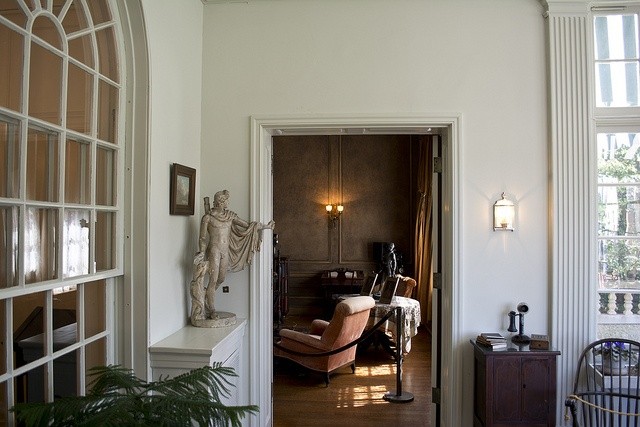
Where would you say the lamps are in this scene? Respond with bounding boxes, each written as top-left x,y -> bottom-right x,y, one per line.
326,205 -> 344,225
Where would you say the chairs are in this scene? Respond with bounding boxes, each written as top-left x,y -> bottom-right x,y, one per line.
565,338 -> 640,426
273,296 -> 375,387
395,274 -> 416,297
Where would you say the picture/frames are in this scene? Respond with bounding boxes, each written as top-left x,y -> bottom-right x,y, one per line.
360,273 -> 378,296
379,277 -> 399,304
169,163 -> 196,215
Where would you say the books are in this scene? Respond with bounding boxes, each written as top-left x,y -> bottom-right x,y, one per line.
475,332 -> 507,350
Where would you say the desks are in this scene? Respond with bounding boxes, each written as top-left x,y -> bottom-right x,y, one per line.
321,270 -> 364,319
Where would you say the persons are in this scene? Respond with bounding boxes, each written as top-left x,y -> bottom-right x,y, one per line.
195,189 -> 276,320
377,242 -> 397,296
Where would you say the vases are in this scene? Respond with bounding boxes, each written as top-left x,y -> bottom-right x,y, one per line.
603,361 -> 629,375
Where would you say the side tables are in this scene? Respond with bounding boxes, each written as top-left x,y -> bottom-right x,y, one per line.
336,294 -> 421,363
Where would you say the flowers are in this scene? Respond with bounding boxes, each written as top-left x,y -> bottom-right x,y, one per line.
593,341 -> 637,362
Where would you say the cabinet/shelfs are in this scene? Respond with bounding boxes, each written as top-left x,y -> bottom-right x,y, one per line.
470,338 -> 560,427
18,320 -> 78,407
148,318 -> 247,426
275,257 -> 289,317
